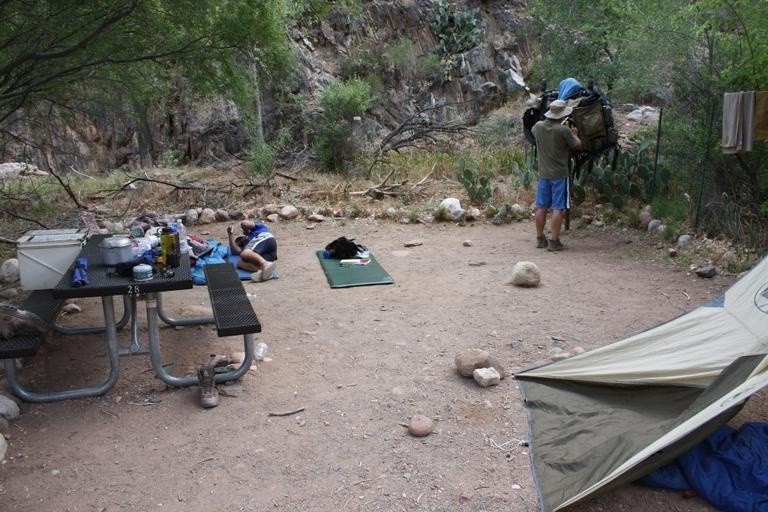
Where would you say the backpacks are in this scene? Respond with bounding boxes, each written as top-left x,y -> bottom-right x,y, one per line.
522,78 -> 623,154
325,236 -> 365,258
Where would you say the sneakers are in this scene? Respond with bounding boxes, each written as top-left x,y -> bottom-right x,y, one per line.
250,259 -> 275,283
0,303 -> 49,340
536,233 -> 569,251
196,351 -> 230,408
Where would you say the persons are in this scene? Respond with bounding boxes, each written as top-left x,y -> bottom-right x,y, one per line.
338,258 -> 373,266
531,100 -> 582,251
226,220 -> 278,281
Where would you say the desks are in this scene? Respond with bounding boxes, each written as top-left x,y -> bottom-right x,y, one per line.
3,233 -> 256,404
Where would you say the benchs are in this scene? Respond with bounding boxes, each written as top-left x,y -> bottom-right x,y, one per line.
1,262 -> 263,404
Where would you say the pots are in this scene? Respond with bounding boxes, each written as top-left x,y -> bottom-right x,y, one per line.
98,237 -> 134,266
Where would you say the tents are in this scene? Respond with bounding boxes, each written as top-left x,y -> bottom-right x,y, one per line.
514,253 -> 768,512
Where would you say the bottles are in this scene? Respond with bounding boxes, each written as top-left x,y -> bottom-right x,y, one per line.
135,235 -> 158,249
248,342 -> 269,364
176,219 -> 187,255
160,227 -> 180,268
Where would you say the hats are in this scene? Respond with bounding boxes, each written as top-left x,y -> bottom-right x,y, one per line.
543,98 -> 573,120
